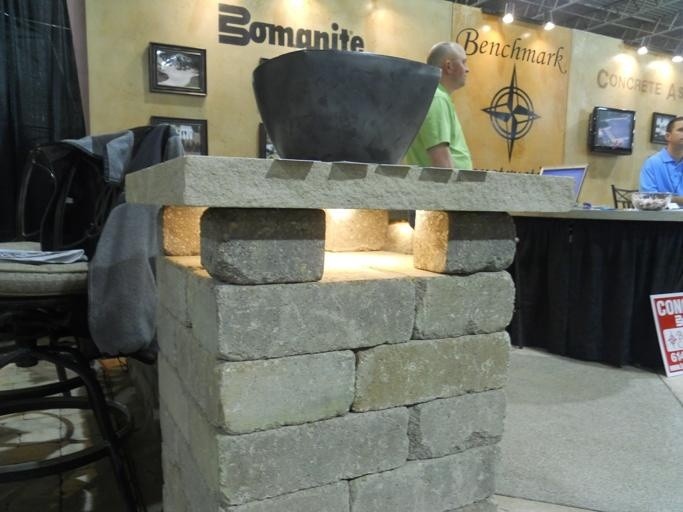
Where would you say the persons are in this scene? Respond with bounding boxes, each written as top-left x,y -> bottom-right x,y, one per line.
403,41 -> 477,171
636,116 -> 682,207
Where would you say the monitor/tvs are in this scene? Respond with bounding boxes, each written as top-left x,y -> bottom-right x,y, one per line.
589,106 -> 635,155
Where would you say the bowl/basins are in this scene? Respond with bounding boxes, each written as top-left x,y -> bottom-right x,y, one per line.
631,191 -> 673,212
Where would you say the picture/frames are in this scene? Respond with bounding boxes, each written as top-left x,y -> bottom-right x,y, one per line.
259,124 -> 277,157
151,116 -> 208,156
148,42 -> 207,95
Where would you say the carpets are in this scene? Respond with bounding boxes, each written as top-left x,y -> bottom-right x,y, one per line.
496,346 -> 682,512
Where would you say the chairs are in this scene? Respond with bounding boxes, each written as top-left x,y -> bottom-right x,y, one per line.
0,125 -> 185,512
611,185 -> 639,209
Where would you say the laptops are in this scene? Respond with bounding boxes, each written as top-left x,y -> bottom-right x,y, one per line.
540,164 -> 589,207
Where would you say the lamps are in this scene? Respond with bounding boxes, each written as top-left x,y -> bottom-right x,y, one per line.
502,3 -> 515,25
543,8 -> 556,32
638,36 -> 652,55
671,42 -> 683,63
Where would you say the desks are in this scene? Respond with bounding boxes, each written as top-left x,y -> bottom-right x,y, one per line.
509,208 -> 682,376
